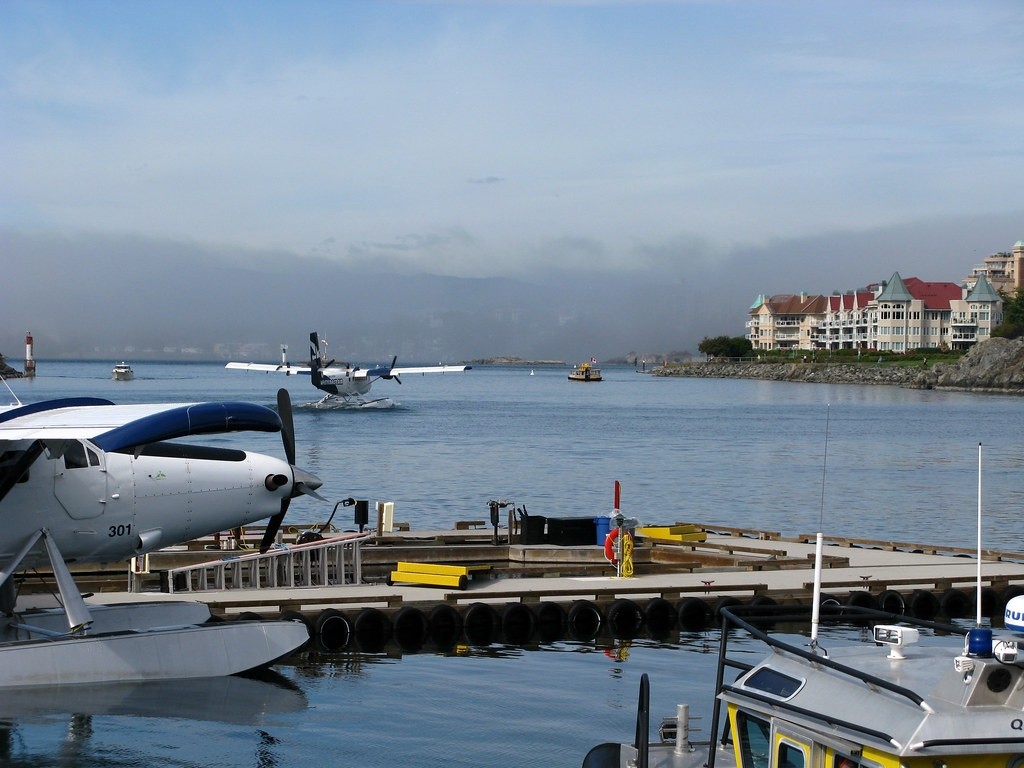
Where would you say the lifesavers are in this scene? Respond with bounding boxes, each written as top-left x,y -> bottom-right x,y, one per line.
604,644 -> 620,659
602,525 -> 635,567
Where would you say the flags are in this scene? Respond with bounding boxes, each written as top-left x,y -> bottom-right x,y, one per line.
590,357 -> 597,363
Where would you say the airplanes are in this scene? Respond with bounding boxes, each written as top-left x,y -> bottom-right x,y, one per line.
0,374 -> 325,696
226,332 -> 476,411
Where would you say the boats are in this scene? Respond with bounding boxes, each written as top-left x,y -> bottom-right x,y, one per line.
578,442 -> 1024,767
567,362 -> 602,382
111,363 -> 134,381
